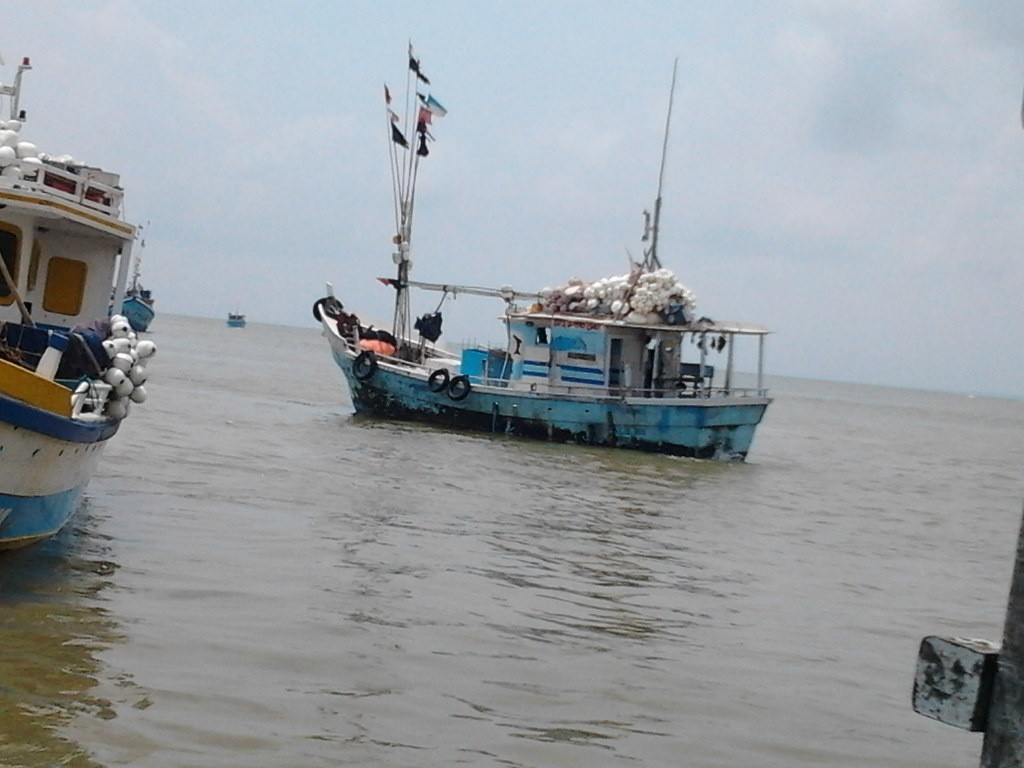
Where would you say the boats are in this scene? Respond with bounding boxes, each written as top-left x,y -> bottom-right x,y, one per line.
313,35 -> 775,468
228,313 -> 244,325
0,53 -> 158,555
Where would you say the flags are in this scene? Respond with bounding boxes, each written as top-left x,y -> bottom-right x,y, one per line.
383,46 -> 448,156
